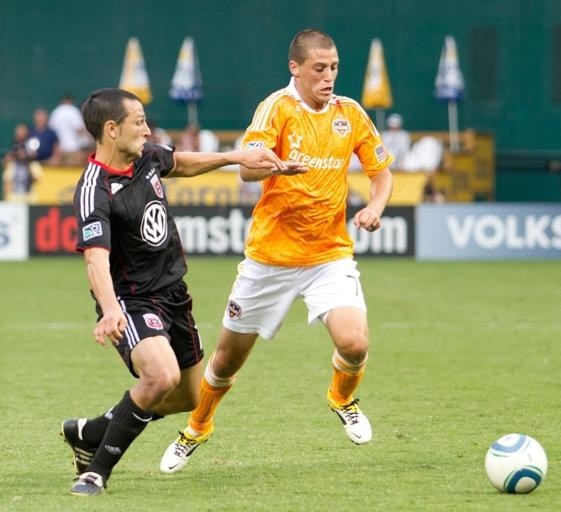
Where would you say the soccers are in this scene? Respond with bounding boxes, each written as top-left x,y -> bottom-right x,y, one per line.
484,434 -> 547,493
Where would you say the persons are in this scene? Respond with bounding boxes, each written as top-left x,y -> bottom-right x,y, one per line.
160,27 -> 397,474
56,87 -> 289,496
381,116 -> 411,169
11,96 -> 89,197
150,125 -> 245,151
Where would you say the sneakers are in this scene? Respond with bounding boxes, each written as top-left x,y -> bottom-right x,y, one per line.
71,472 -> 107,496
60,418 -> 97,475
160,427 -> 215,473
327,384 -> 373,445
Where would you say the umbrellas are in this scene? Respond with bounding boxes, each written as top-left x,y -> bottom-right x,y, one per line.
359,38 -> 395,127
117,37 -> 154,105
434,34 -> 467,151
165,36 -> 206,134
